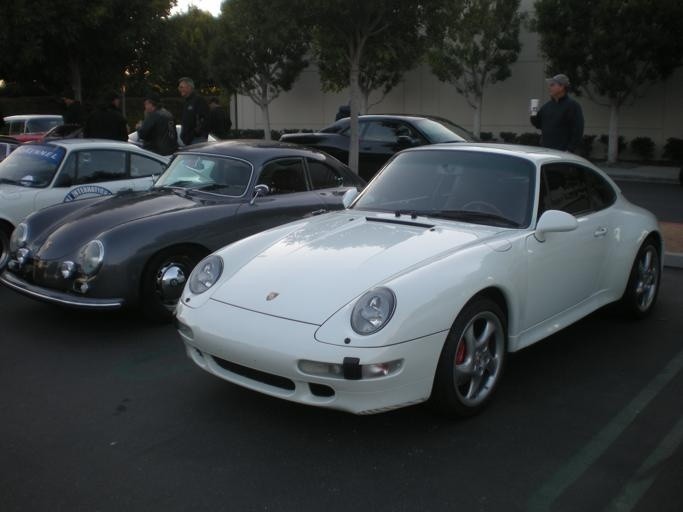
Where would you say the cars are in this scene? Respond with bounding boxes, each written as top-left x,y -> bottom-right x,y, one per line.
0,138 -> 369,325
128,124 -> 218,178
0,114 -> 64,162
1,138 -> 216,250
278,116 -> 481,184
171,150 -> 665,420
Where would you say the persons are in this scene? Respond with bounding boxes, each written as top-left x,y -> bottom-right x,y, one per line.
335,98 -> 362,122
177,77 -> 215,145
529,74 -> 585,154
207,98 -> 233,140
84,90 -> 129,142
61,89 -> 84,125
134,94 -> 178,158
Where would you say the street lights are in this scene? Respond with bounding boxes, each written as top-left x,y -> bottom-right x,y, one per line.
120,80 -> 127,119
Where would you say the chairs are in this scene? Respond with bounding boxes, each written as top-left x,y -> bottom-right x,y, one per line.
310,162 -> 329,185
442,168 -> 487,212
224,166 -> 248,193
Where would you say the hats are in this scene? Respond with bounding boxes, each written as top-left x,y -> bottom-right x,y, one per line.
544,73 -> 570,88
143,91 -> 161,102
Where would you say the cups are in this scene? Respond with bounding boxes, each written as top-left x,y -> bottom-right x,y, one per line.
529,98 -> 539,116
